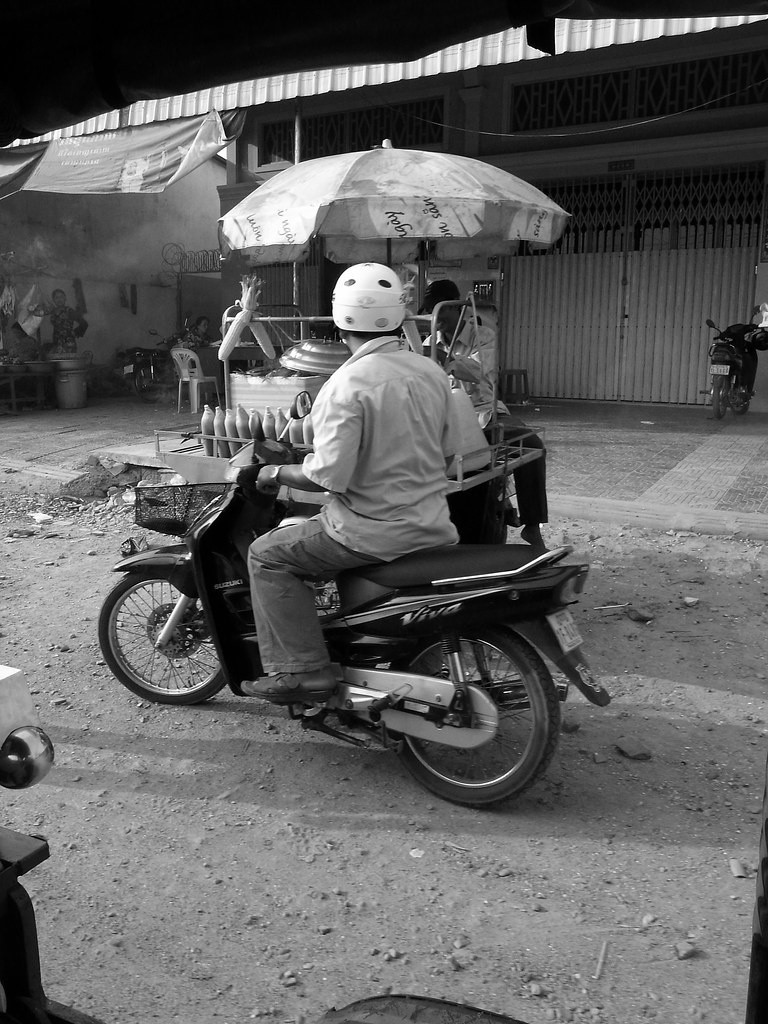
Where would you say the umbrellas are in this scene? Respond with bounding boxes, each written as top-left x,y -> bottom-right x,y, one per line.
218,139 -> 572,269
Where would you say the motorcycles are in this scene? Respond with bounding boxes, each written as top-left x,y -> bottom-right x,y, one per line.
120,310 -> 198,404
699,304 -> 768,420
97,390 -> 611,810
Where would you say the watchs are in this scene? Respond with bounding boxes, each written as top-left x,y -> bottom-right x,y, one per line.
270,465 -> 284,486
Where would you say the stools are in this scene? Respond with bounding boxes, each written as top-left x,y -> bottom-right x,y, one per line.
501,369 -> 529,404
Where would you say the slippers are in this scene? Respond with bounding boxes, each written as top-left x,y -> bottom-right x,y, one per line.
240,670 -> 334,700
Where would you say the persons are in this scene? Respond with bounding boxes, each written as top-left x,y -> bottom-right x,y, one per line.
416,280 -> 549,548
8,288 -> 88,356
240,262 -> 460,700
183,317 -> 221,354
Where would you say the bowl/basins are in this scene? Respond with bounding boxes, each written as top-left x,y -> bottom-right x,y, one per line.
0,358 -> 84,372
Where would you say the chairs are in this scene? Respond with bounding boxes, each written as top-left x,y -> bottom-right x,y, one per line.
170,348 -> 222,414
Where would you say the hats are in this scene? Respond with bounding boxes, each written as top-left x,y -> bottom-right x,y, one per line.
417,280 -> 461,314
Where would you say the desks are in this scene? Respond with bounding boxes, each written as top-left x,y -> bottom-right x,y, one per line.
197,344 -> 290,404
0,372 -> 56,414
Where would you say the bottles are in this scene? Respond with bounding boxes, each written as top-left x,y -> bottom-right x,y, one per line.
201,405 -> 314,458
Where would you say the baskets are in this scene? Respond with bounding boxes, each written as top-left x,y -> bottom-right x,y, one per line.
133,479 -> 237,538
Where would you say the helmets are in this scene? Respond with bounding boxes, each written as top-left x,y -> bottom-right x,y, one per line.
332,262 -> 407,333
